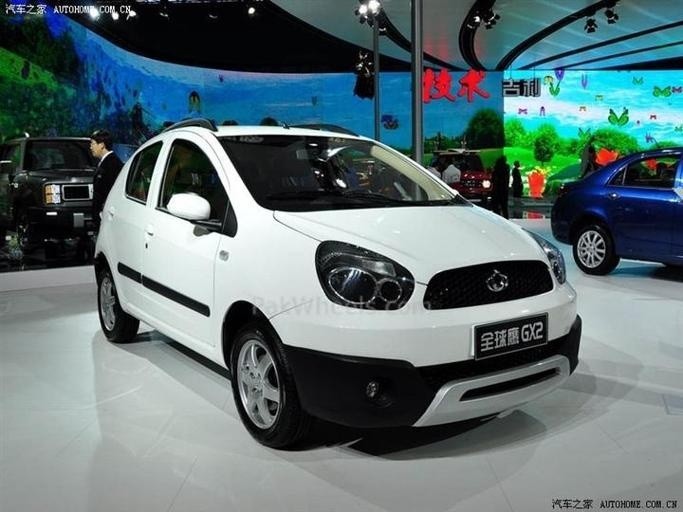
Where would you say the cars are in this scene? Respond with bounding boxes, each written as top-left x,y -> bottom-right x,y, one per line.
548,143 -> 682,278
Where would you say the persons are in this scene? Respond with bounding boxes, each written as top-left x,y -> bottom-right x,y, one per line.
490,156 -> 522,220
90,130 -> 124,227
426,160 -> 461,184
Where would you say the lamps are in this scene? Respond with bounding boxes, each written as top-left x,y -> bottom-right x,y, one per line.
354,0 -> 388,35
468,5 -> 501,29
584,7 -> 619,33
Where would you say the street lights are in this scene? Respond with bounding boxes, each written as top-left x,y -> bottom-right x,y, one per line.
357,0 -> 387,142
